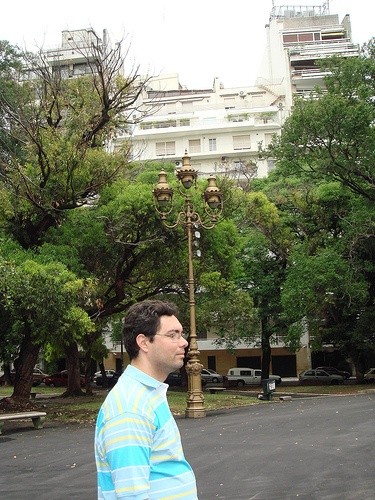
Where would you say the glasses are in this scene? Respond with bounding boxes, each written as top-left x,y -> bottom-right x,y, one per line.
150,332 -> 187,343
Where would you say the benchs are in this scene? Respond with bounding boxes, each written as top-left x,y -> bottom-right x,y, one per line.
206,387 -> 226,394
0,411 -> 47,434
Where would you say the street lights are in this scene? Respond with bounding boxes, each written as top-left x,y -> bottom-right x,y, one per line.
151,147 -> 224,420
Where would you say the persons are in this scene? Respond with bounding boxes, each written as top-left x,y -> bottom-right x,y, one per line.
95,299 -> 198,500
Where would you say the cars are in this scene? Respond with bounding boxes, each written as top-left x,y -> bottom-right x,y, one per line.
200,368 -> 223,383
44,370 -> 86,388
299,369 -> 343,385
163,372 -> 207,387
363,368 -> 375,383
0,368 -> 51,388
94,369 -> 115,379
316,366 -> 350,380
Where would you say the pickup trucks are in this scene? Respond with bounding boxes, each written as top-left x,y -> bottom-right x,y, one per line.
226,368 -> 282,388
